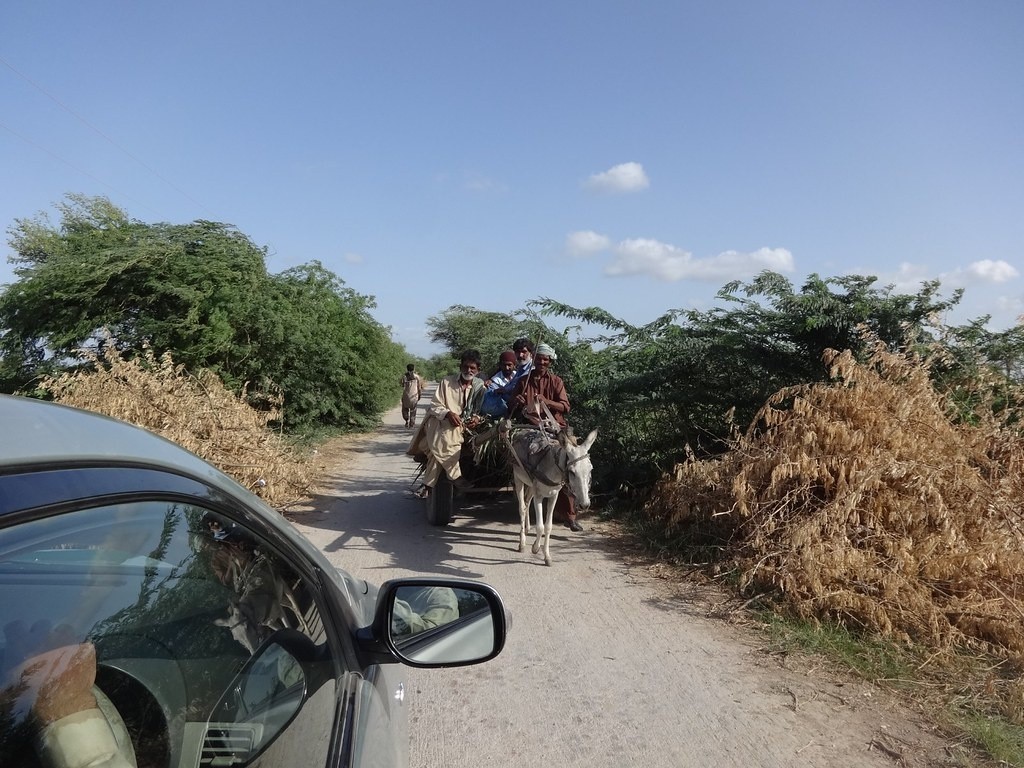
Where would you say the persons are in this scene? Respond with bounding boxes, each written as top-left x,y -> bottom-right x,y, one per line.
392,586 -> 460,635
399,364 -> 422,428
0,624 -> 136,768
203,512 -> 271,589
407,338 -> 583,533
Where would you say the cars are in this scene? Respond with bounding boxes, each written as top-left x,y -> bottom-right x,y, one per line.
0,386 -> 516,767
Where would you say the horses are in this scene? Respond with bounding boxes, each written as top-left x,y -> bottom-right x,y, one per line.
511,426 -> 597,567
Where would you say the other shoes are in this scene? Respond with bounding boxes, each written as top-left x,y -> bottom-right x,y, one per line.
448,476 -> 475,489
564,519 -> 583,532
414,486 -> 428,499
405,421 -> 408,428
410,423 -> 413,427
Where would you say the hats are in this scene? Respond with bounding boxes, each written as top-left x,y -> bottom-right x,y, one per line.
534,343 -> 557,360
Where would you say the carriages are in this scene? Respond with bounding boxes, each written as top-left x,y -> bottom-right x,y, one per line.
425,418 -> 601,568
